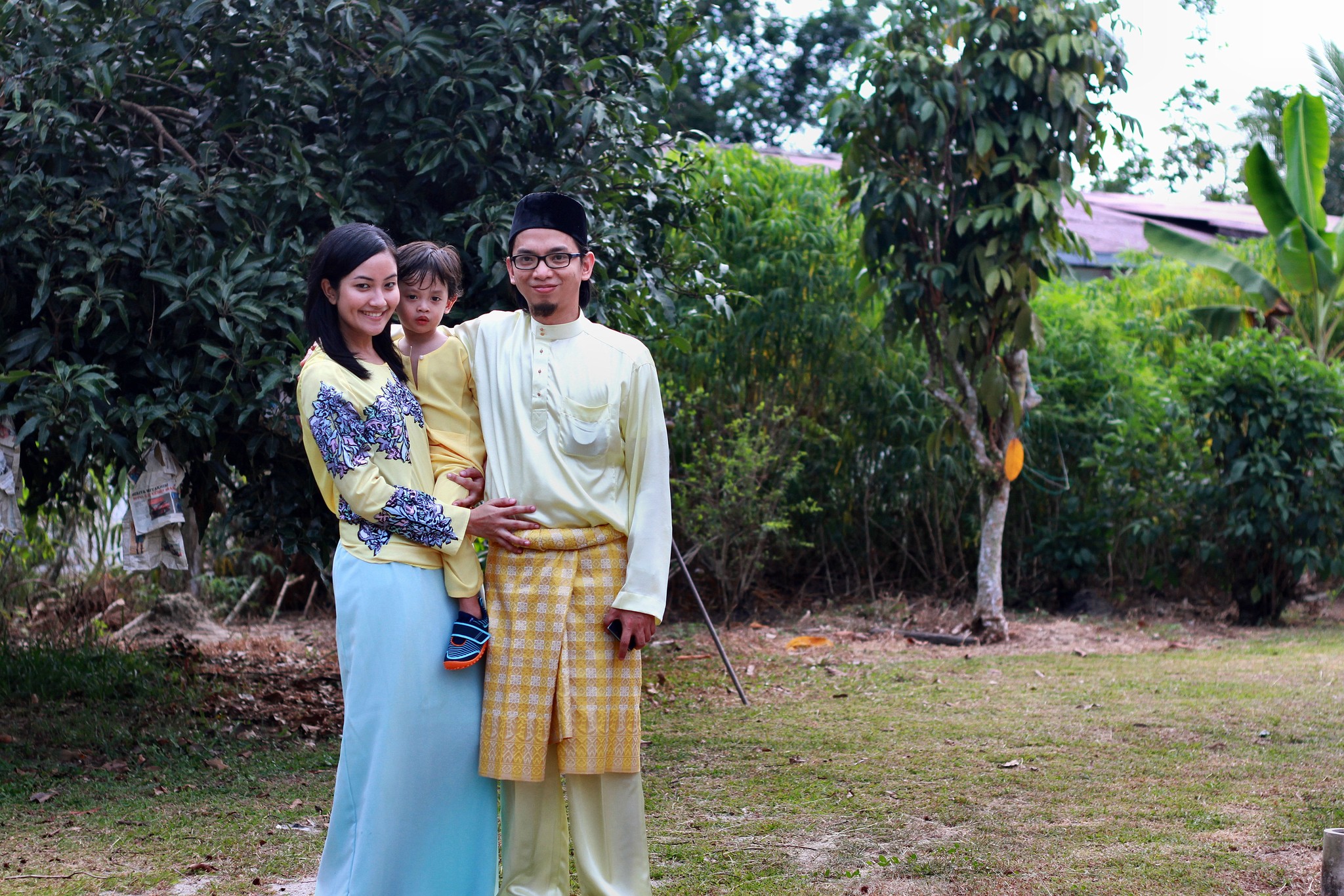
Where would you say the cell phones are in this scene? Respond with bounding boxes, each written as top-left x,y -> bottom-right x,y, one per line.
606,618 -> 637,652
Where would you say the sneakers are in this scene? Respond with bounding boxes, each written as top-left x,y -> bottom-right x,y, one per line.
443,597 -> 490,670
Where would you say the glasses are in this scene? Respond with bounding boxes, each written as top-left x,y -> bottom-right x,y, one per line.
511,252 -> 583,270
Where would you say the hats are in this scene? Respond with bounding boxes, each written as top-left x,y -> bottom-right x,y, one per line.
508,192 -> 591,309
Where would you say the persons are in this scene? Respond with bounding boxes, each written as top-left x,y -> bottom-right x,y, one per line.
300,190 -> 680,896
297,222 -> 544,896
